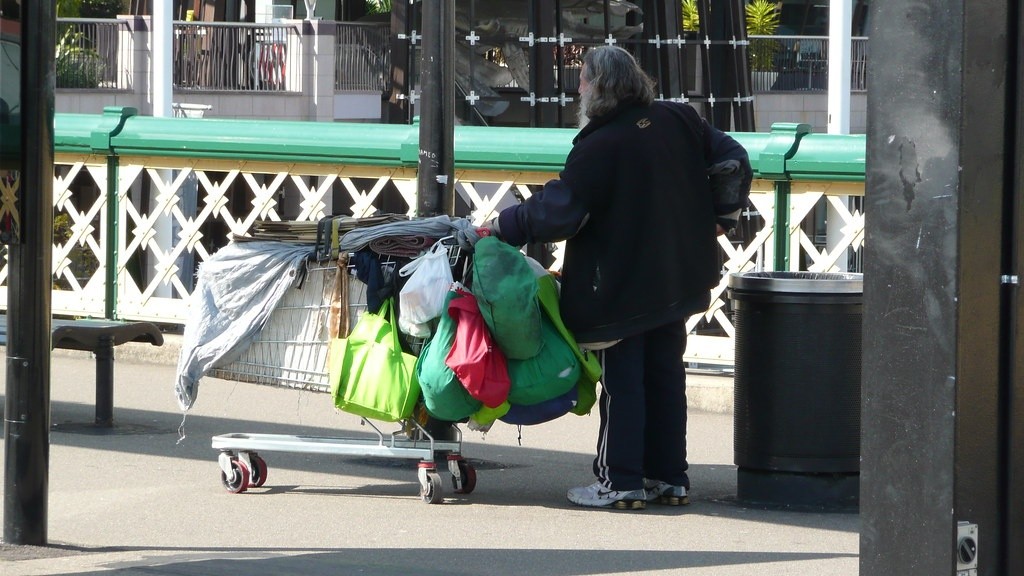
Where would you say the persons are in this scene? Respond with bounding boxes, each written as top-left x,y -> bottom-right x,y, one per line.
481,45 -> 753,511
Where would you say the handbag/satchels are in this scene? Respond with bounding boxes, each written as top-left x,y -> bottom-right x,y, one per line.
330,234 -> 602,432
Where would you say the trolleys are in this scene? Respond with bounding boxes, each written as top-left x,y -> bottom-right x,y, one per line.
205,228 -> 490,504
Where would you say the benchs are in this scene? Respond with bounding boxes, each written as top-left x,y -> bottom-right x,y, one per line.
0,314 -> 164,437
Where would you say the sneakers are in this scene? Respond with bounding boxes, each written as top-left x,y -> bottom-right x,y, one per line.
567,481 -> 647,511
642,477 -> 690,507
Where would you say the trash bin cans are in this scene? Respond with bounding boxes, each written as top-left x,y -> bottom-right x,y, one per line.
728,270 -> 863,513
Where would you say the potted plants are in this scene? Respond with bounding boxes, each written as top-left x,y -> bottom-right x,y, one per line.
682,0 -> 700,40
744,0 -> 782,91
